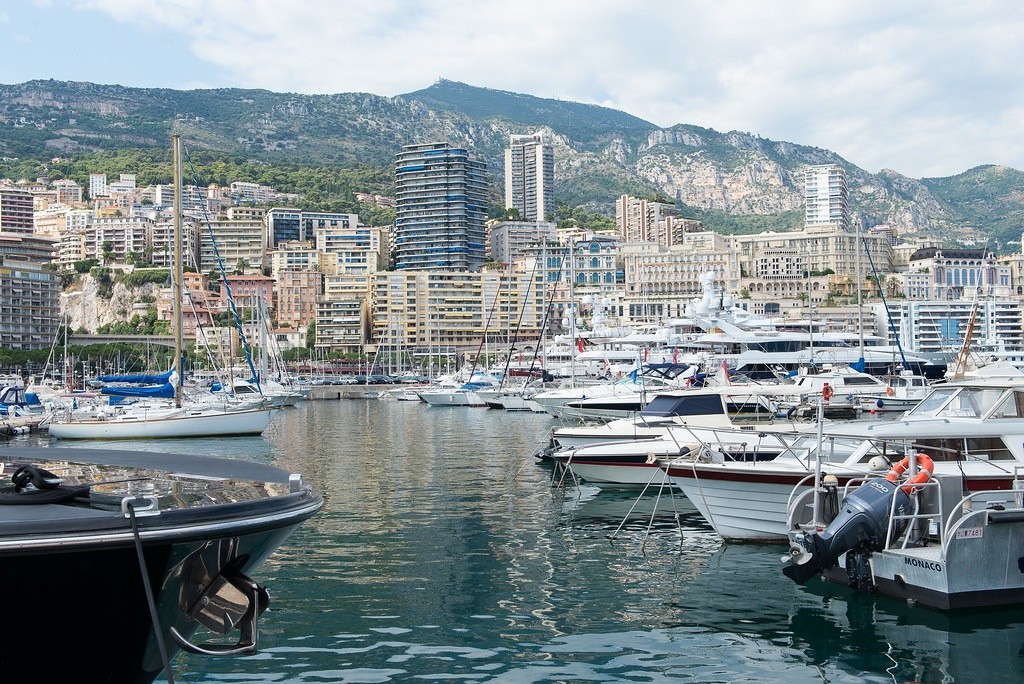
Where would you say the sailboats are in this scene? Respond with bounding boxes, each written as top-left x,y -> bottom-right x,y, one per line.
0,133 -> 1024,491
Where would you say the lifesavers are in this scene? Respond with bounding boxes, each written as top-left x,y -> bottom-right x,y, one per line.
13,430 -> 17,435
886,453 -> 934,494
822,385 -> 833,397
7,428 -> 10,436
597,376 -> 608,380
887,388 -> 893,396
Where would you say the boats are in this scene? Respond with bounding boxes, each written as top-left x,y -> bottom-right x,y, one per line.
780,426 -> 1024,611
644,377 -> 1023,543
0,445 -> 326,684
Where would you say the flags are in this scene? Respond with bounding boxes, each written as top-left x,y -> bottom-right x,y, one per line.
721,361 -> 730,382
518,353 -> 524,363
578,336 -> 587,353
628,370 -> 637,385
673,347 -> 680,364
538,357 -> 543,368
